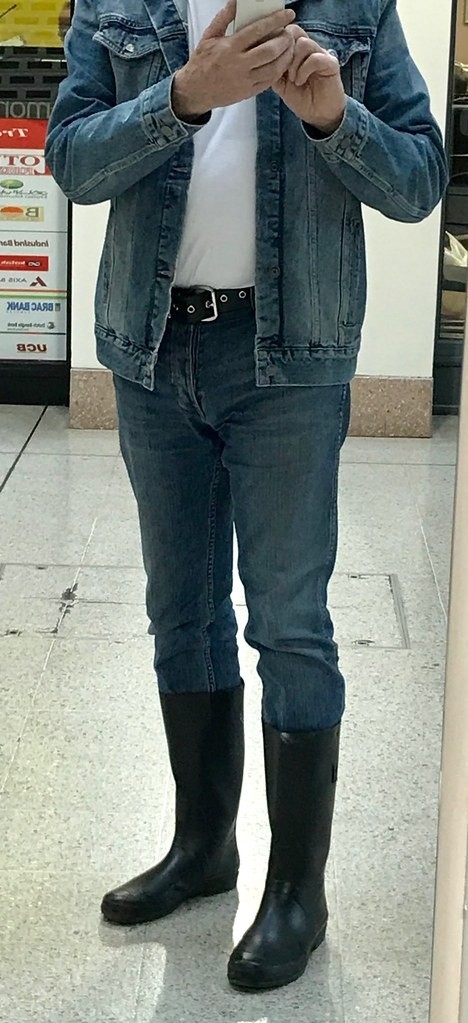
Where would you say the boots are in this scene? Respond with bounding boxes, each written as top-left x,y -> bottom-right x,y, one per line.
228,725 -> 340,994
102,676 -> 244,926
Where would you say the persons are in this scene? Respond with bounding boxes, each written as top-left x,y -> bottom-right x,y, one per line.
43,1 -> 453,993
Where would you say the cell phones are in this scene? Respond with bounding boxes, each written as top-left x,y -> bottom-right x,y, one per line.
233,0 -> 285,45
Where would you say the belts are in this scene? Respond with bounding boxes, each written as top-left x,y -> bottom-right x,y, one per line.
169,284 -> 256,328
